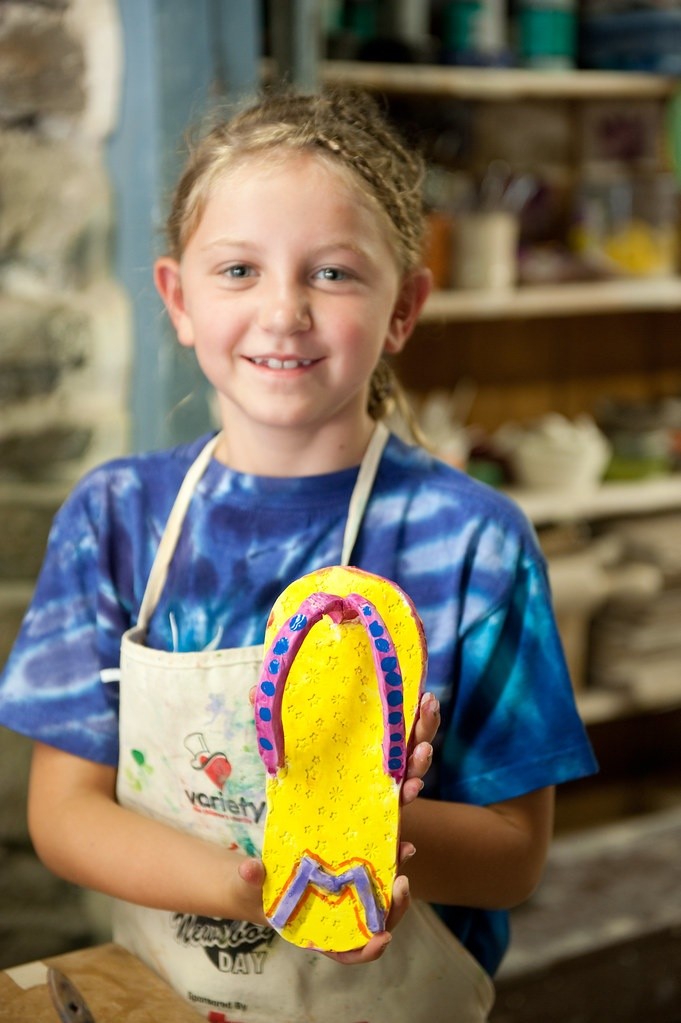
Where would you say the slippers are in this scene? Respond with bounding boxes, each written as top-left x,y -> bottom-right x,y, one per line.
255,565 -> 428,951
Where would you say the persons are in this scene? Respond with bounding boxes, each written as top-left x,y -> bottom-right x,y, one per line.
0,82 -> 601,1022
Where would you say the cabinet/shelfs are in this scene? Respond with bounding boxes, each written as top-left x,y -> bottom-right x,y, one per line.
259,1 -> 680,851
